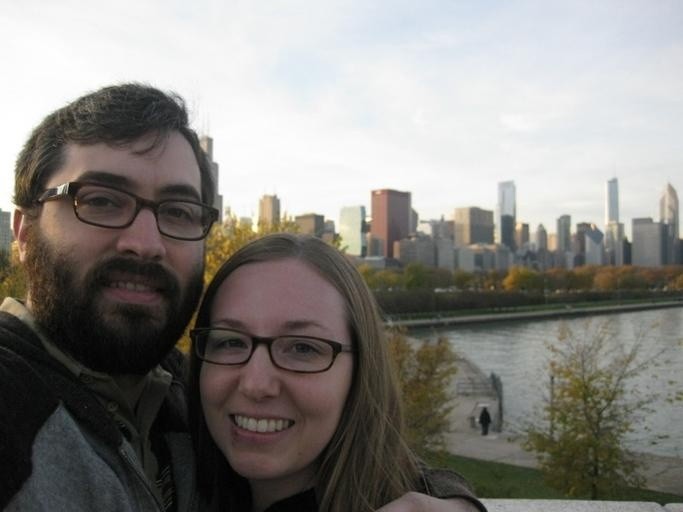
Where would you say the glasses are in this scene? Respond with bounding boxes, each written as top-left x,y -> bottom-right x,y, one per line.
38,182 -> 219,242
189,327 -> 355,374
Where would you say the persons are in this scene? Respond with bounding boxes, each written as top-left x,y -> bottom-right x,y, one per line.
1,81 -> 491,511
184,233 -> 430,512
477,405 -> 491,436
